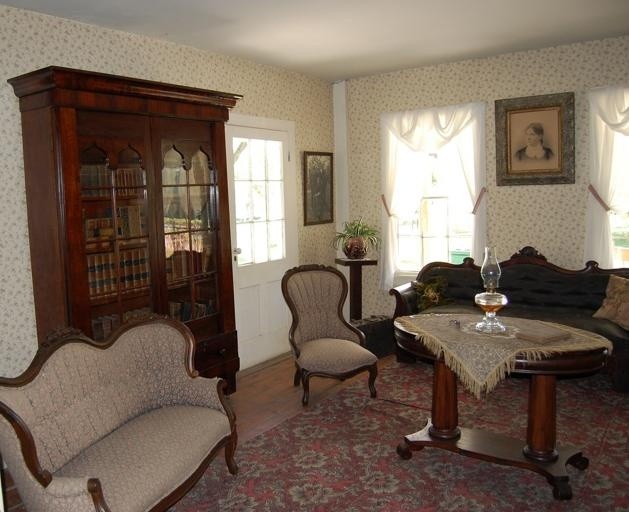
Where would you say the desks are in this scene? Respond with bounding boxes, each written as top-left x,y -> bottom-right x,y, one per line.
394,313 -> 613,501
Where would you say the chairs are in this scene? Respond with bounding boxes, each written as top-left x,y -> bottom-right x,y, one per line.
281,264 -> 378,407
0,314 -> 239,512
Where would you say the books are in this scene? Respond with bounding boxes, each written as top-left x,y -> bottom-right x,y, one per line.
167,232 -> 215,321
80,166 -> 143,198
85,248 -> 151,299
92,308 -> 155,342
84,205 -> 142,248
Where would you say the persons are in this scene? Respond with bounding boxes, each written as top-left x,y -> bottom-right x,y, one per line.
514,122 -> 554,162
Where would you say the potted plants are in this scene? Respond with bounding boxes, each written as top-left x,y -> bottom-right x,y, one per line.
329,216 -> 382,260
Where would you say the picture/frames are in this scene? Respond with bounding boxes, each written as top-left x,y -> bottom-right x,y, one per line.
494,91 -> 575,186
303,151 -> 334,226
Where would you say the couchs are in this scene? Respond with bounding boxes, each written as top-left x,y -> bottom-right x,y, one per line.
389,245 -> 629,393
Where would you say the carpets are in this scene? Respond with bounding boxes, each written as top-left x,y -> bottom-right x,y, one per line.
166,361 -> 628,512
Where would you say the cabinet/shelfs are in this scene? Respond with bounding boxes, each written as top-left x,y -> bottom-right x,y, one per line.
7,65 -> 243,396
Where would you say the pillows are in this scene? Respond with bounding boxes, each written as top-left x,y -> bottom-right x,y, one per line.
592,274 -> 629,331
411,275 -> 454,314
